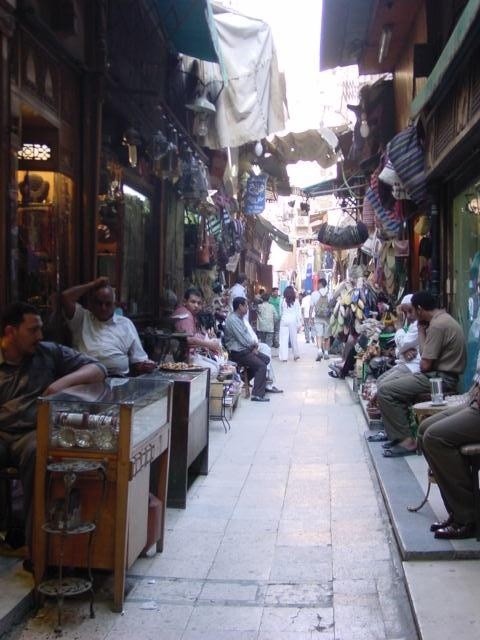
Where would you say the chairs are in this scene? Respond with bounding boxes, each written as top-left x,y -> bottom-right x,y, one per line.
207,382 -> 234,433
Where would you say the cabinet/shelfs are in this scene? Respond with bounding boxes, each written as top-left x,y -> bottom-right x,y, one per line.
131,366 -> 212,511
34,457 -> 107,634
30,373 -> 176,617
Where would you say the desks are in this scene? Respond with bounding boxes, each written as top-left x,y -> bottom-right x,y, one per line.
406,396 -> 461,519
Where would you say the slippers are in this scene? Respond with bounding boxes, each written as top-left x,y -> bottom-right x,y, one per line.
384,438 -> 401,448
382,446 -> 417,457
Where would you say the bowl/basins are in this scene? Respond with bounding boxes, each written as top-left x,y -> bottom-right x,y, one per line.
57,427 -> 91,448
92,425 -> 119,452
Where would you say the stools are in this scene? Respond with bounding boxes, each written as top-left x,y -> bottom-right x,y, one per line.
411,391 -> 461,457
235,361 -> 254,400
461,442 -> 479,545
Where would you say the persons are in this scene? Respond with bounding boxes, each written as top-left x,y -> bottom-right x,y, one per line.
169,273 -> 283,402
280,278 -> 334,363
61,276 -> 157,383
0,303 -> 110,555
328,269 -> 467,459
416,361 -> 480,539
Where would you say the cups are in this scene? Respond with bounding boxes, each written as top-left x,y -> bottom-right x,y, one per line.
428,377 -> 444,403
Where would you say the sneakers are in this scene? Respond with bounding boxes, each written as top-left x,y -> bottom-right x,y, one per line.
323,355 -> 329,360
317,352 -> 324,360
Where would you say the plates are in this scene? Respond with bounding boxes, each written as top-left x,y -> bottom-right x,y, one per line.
157,365 -> 201,373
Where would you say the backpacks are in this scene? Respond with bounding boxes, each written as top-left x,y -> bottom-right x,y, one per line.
315,288 -> 332,318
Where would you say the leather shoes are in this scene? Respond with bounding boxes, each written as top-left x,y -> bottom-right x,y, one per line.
251,394 -> 270,402
434,522 -> 479,539
430,512 -> 459,531
266,386 -> 283,393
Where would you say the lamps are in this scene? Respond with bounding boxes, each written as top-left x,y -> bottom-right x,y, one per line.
121,92 -> 143,168
358,42 -> 373,138
253,137 -> 264,159
377,25 -> 394,64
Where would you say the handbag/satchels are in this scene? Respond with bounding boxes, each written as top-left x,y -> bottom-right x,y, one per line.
192,354 -> 219,380
318,223 -> 410,284
195,229 -> 246,319
